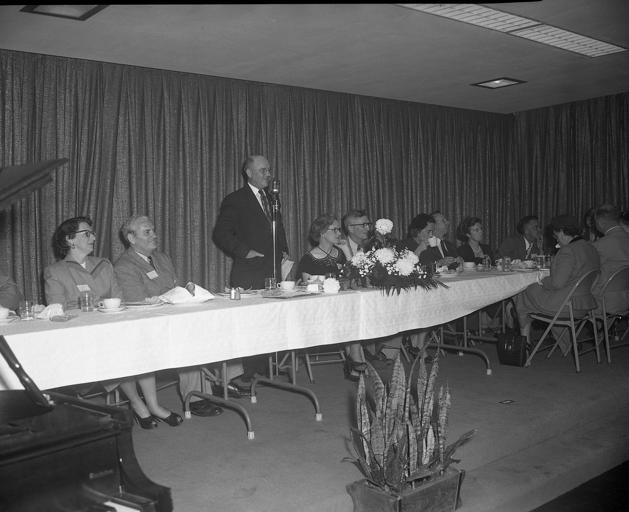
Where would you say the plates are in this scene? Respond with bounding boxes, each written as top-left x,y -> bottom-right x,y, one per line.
127,301 -> 166,310
0,316 -> 17,324
218,293 -> 254,298
511,268 -> 534,272
98,307 -> 127,313
540,267 -> 552,271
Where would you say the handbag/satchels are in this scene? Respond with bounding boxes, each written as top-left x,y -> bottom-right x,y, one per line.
495,325 -> 530,366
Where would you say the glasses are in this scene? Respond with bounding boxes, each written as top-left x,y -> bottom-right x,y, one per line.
349,223 -> 371,228
326,228 -> 342,232
251,168 -> 272,175
69,230 -> 96,238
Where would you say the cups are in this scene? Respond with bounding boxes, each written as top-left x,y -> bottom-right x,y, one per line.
97,298 -> 121,309
79,290 -> 95,312
464,261 -> 475,267
310,274 -> 325,284
19,300 -> 35,322
0,306 -> 14,318
264,277 -> 276,293
277,281 -> 296,290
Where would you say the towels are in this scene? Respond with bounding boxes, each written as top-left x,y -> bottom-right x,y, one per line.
160,282 -> 216,303
38,303 -> 62,319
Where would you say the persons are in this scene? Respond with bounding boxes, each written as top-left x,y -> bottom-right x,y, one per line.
42,216 -> 183,429
1,270 -> 21,319
493,216 -> 543,263
114,217 -> 256,416
516,214 -> 600,365
399,214 -> 440,363
456,216 -> 497,268
210,154 -> 290,291
297,212 -> 369,381
590,205 -> 629,314
342,210 -> 371,262
432,211 -> 464,273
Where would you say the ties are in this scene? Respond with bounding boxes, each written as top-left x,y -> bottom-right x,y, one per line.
148,256 -> 156,273
440,240 -> 447,258
527,242 -> 532,259
258,189 -> 272,226
358,246 -> 361,251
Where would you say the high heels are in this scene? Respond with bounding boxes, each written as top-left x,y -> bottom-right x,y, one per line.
343,361 -> 365,382
346,355 -> 366,373
128,404 -> 158,430
153,406 -> 183,427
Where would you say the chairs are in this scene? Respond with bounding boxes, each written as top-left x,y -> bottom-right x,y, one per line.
526,267 -> 606,375
562,267 -> 629,364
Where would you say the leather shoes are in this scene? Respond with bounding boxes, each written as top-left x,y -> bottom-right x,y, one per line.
212,383 -> 256,398
410,346 -> 428,363
571,343 -> 580,368
415,346 -> 433,362
184,399 -> 223,417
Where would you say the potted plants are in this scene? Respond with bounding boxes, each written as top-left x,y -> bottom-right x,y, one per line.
344,326 -> 472,510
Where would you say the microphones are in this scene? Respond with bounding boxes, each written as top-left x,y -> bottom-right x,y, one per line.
268,178 -> 281,206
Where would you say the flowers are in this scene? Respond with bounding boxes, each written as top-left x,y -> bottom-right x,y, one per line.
345,216 -> 448,296
